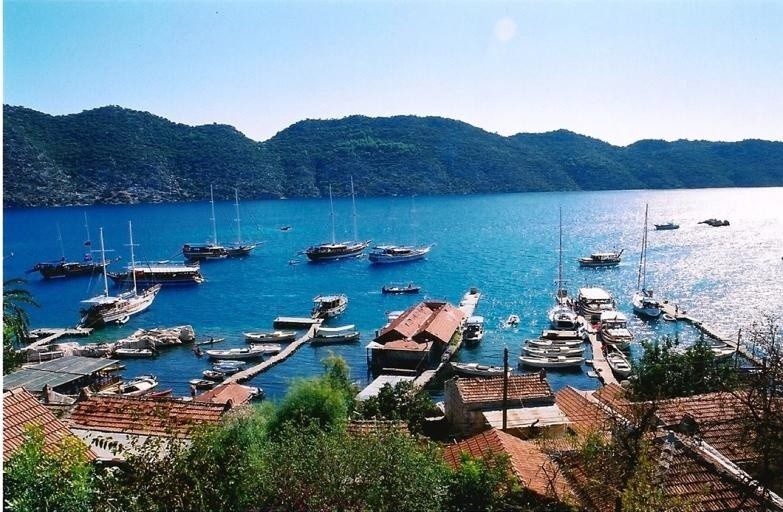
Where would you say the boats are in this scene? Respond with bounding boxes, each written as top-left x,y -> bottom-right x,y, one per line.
658,342 -> 740,365
698,218 -> 730,228
505,314 -> 519,329
516,200 -> 680,376
447,360 -> 514,378
463,315 -> 485,344
